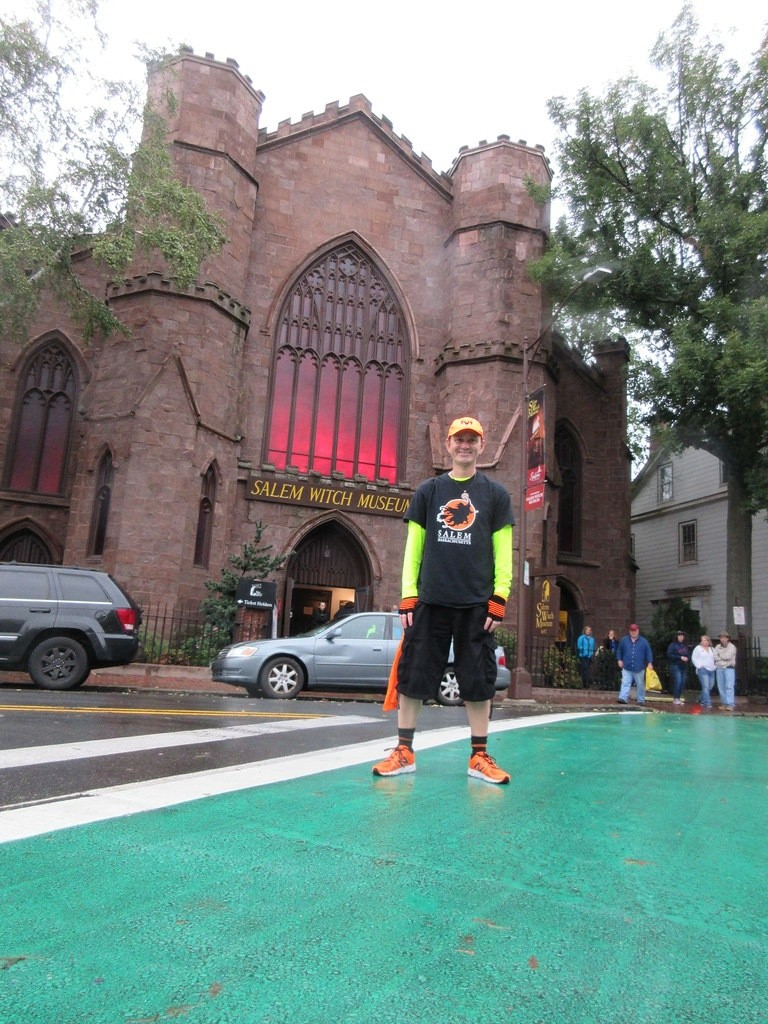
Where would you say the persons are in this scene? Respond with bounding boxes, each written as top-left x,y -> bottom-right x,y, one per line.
372,416 -> 511,784
715,631 -> 736,711
691,635 -> 716,708
603,629 -> 619,689
615,624 -> 653,704
577,626 -> 595,688
312,602 -> 329,627
666,631 -> 691,705
336,601 -> 354,617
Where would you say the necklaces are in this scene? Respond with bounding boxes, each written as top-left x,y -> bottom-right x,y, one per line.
453,476 -> 475,500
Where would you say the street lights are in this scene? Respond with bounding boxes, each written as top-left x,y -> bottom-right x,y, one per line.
506,266 -> 614,700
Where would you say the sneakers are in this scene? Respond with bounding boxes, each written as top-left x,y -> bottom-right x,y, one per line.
467,751 -> 510,784
372,744 -> 416,776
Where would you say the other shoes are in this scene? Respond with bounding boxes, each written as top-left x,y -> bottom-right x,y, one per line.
697,701 -> 712,708
673,697 -> 683,705
719,705 -> 734,711
617,699 -> 626,704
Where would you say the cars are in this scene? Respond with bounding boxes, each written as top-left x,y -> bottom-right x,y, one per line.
211,610 -> 515,707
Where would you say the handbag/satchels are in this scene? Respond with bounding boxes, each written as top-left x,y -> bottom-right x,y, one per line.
645,667 -> 662,691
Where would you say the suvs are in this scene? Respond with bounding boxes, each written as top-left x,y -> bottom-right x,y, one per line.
0,561 -> 145,692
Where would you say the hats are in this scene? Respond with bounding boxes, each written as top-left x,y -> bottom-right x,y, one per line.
630,623 -> 640,631
677,631 -> 684,635
718,631 -> 730,637
448,417 -> 484,439
